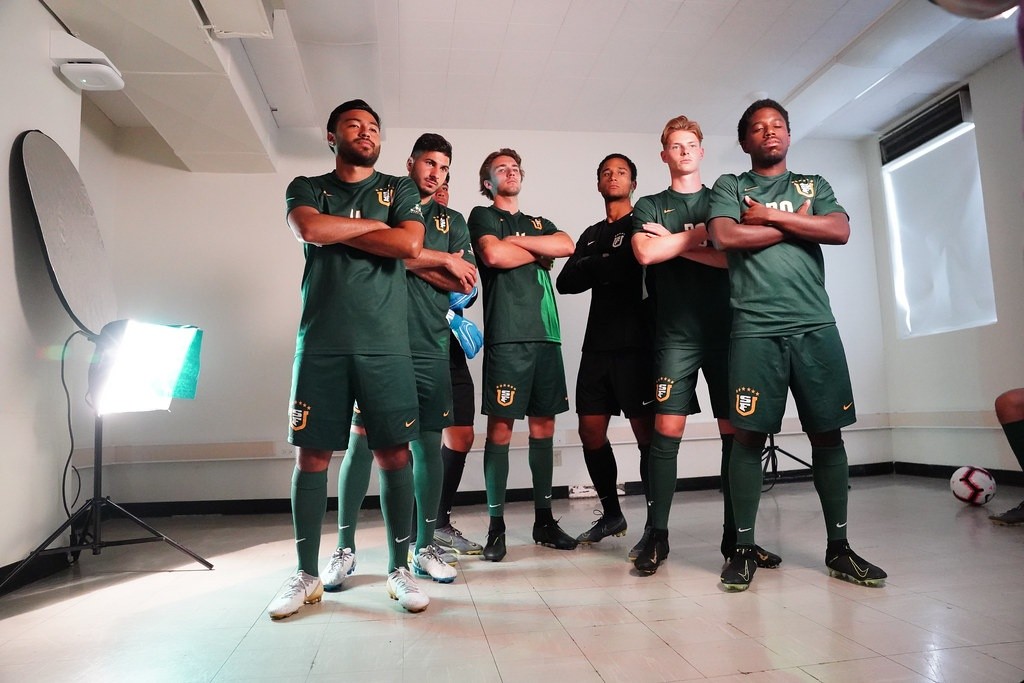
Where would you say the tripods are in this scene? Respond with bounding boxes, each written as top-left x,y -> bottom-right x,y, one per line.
762,434 -> 852,489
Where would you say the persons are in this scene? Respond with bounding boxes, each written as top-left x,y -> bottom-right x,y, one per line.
705,98 -> 888,592
320,133 -> 483,591
268,99 -> 430,620
556,154 -> 654,560
469,148 -> 580,562
988,388 -> 1024,526
629,115 -> 782,572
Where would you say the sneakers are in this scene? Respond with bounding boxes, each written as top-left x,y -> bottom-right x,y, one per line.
576,509 -> 627,545
412,545 -> 457,584
321,547 -> 357,592
825,538 -> 887,586
386,567 -> 430,612
628,524 -> 650,561
484,531 -> 507,562
407,538 -> 458,567
720,524 -> 782,569
269,570 -> 324,620
433,521 -> 484,555
988,501 -> 1024,526
532,516 -> 579,550
720,545 -> 758,591
634,527 -> 670,574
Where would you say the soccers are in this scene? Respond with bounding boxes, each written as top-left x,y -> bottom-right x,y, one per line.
949,466 -> 998,506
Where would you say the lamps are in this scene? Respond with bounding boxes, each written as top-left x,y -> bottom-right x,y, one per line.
84,318 -> 198,418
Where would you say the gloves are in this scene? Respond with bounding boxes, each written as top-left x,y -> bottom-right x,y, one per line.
450,286 -> 478,309
445,309 -> 482,359
